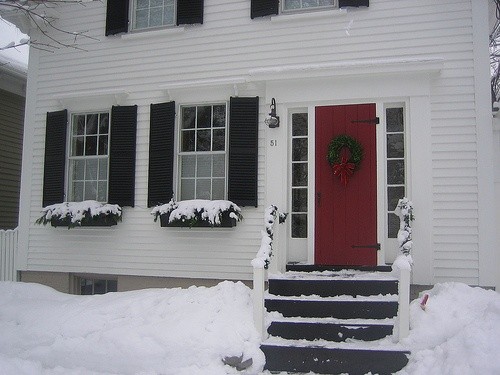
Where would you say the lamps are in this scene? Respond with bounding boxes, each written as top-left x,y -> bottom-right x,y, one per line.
265,98 -> 280,129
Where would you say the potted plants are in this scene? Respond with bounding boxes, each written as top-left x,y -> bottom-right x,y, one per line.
33,201 -> 124,228
151,198 -> 244,229
